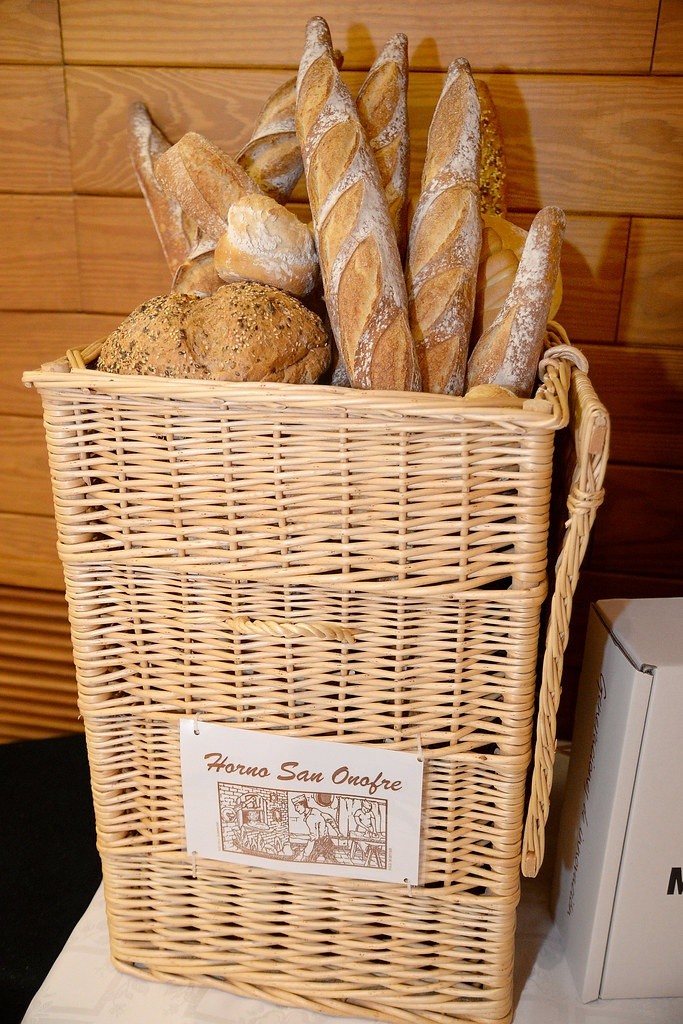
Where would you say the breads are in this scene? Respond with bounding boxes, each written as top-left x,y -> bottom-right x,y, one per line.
93,16 -> 567,407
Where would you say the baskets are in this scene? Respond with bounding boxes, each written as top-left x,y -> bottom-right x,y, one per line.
21,320 -> 609,1024
